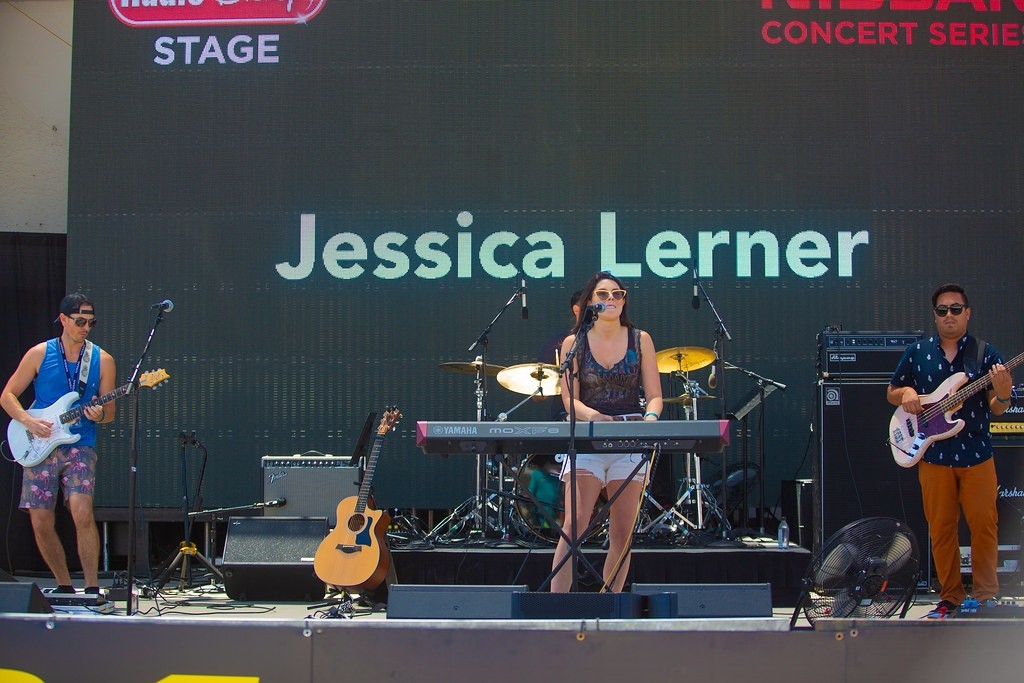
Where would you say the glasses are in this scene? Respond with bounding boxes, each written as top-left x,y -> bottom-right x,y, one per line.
69,316 -> 98,327
593,290 -> 626,300
934,303 -> 968,318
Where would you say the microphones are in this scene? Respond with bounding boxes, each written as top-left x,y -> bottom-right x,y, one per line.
274,497 -> 287,507
708,339 -> 718,389
692,258 -> 700,309
521,278 -> 528,320
588,302 -> 606,312
151,300 -> 174,313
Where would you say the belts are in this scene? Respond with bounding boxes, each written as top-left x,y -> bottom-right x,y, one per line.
612,416 -> 644,422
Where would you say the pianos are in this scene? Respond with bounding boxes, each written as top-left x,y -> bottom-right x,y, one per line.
415,420 -> 731,592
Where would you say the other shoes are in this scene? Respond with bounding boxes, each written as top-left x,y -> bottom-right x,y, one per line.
927,601 -> 955,619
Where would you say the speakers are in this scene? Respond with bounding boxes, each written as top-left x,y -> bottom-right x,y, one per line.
0,582 -> 54,613
221,516 -> 331,602
512,591 -> 649,620
957,434 -> 1024,547
261,456 -> 368,528
810,380 -> 934,594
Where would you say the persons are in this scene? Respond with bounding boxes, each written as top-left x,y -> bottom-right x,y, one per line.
550,271 -> 665,594
0,294 -> 117,606
530,288 -> 596,424
886,284 -> 1012,619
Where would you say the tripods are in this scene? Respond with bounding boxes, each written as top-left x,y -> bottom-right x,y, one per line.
421,366 -> 545,542
646,358 -> 736,541
145,430 -> 224,598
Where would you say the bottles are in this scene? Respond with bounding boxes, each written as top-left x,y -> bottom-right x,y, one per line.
778,517 -> 789,549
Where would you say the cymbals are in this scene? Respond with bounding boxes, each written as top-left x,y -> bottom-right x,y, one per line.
496,362 -> 565,397
655,345 -> 717,373
662,393 -> 719,406
438,360 -> 508,377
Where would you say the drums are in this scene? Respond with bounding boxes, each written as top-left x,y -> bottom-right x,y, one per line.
514,448 -> 625,545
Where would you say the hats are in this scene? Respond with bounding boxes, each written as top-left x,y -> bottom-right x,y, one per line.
53,292 -> 95,324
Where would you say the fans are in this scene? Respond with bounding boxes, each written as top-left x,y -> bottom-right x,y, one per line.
790,516 -> 922,633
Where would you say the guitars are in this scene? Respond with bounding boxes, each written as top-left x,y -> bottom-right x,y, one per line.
312,404 -> 403,593
888,345 -> 1024,469
7,368 -> 170,468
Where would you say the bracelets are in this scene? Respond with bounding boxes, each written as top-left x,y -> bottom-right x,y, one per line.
996,395 -> 1012,404
644,412 -> 659,421
94,410 -> 105,422
